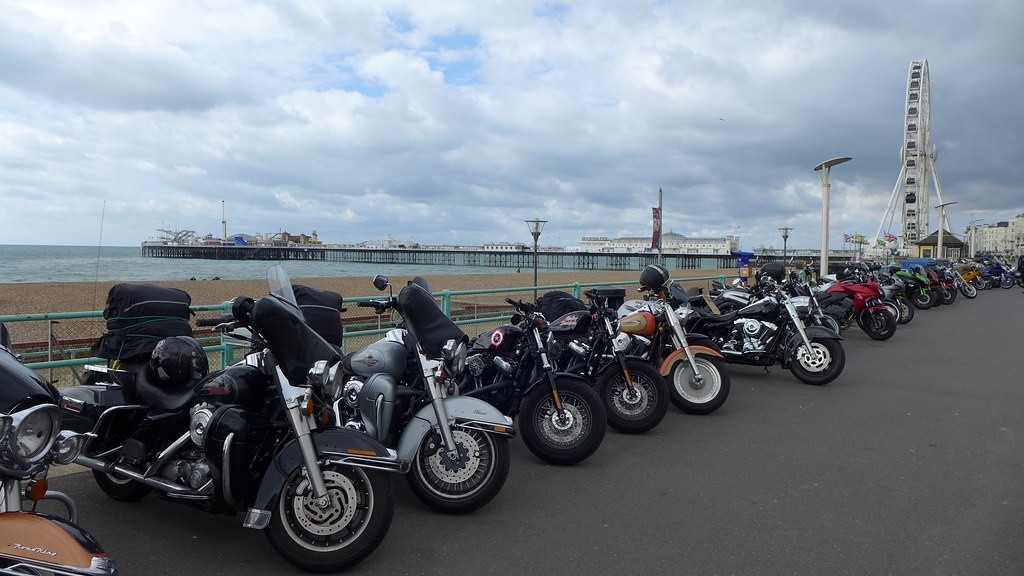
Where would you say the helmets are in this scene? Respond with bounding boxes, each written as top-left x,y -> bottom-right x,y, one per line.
148,336 -> 209,391
640,264 -> 670,293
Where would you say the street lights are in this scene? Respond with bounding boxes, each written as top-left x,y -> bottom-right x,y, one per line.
815,157 -> 854,275
778,227 -> 794,266
936,202 -> 959,258
524,218 -> 549,300
969,219 -> 985,258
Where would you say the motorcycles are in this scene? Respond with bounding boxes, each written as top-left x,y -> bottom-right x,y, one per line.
0,256 -> 1024,576
